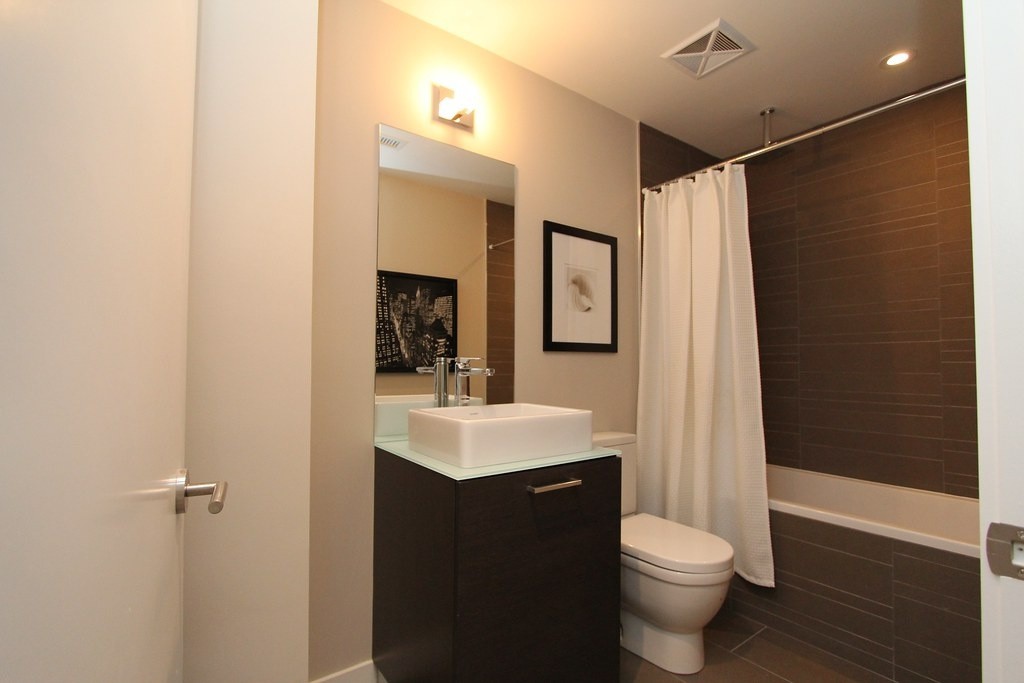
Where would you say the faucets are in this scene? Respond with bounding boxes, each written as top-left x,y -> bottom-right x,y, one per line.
454,356 -> 496,407
416,357 -> 454,407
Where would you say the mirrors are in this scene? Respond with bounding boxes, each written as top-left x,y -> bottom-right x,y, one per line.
373,122 -> 516,442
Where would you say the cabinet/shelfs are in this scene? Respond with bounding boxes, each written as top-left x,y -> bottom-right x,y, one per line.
372,454 -> 622,683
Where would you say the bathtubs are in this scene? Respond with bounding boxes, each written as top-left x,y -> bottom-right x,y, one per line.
723,463 -> 982,682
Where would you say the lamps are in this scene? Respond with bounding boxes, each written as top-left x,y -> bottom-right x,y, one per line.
439,70 -> 482,130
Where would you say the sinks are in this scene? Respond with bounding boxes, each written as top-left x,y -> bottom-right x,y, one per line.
407,402 -> 593,470
375,394 -> 484,444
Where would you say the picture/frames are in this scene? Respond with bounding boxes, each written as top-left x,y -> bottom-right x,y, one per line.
543,220 -> 619,354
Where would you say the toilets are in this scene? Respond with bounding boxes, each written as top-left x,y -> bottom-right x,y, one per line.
592,431 -> 735,675
